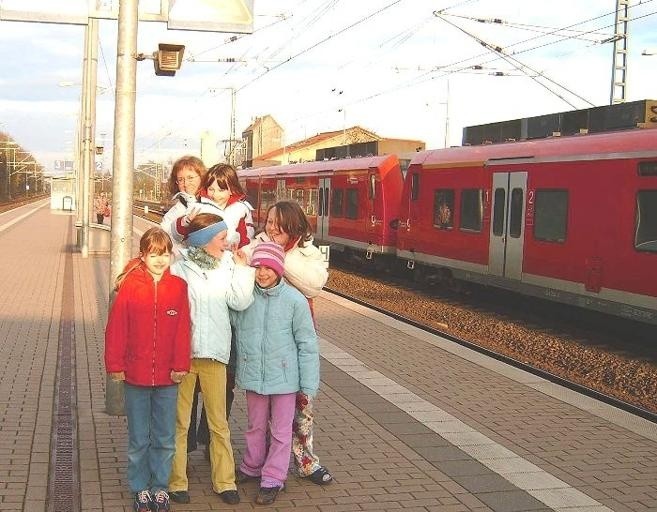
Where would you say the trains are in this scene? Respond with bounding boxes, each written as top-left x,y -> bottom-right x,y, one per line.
159,127 -> 656,337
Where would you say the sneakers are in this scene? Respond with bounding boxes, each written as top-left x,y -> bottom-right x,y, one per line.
168,490 -> 191,505
256,486 -> 281,505
229,470 -> 252,484
219,490 -> 241,506
134,488 -> 153,512
302,466 -> 333,486
150,488 -> 171,512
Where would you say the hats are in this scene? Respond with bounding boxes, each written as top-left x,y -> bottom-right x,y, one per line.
249,241 -> 286,276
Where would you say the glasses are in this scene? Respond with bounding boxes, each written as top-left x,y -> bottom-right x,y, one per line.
176,175 -> 200,185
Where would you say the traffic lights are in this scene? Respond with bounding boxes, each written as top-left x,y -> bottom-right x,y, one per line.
96,146 -> 104,155
157,41 -> 186,71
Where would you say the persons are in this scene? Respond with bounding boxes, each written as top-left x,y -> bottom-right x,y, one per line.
95,191 -> 109,224
